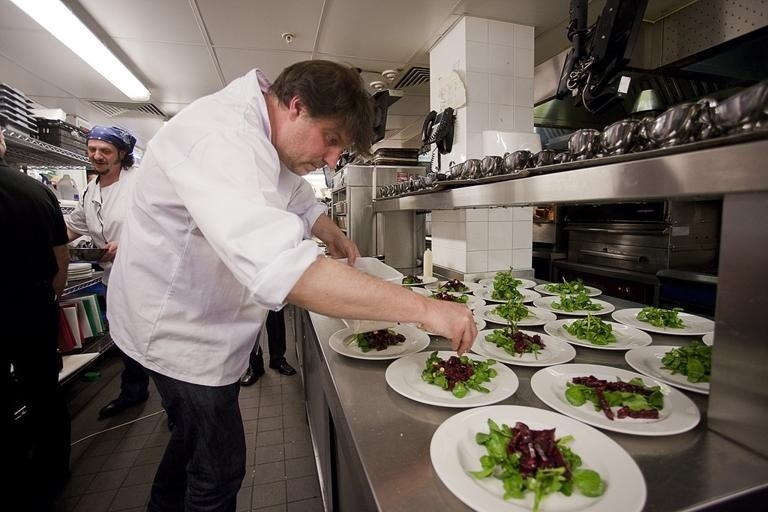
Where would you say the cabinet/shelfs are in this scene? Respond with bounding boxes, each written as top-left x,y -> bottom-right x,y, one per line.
371,127 -> 768,457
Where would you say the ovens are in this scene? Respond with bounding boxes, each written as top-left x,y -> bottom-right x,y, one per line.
549,201 -> 721,278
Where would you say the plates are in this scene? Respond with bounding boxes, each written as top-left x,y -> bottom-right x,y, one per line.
472,289 -> 543,303
469,329 -> 577,370
531,297 -> 615,315
67,263 -> 92,281
328,325 -> 431,368
624,345 -> 709,397
533,278 -> 602,298
423,280 -> 484,294
700,333 -> 713,346
611,306 -> 716,336
528,364 -> 700,438
426,405 -> 649,512
543,319 -> 653,349
400,286 -> 434,297
472,304 -> 557,325
398,276 -> 439,287
383,350 -> 520,409
476,278 -> 537,289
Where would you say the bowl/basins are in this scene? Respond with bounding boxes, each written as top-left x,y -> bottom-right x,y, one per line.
373,76 -> 767,201
69,248 -> 109,261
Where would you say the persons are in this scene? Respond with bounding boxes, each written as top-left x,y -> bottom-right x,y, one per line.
0,127 -> 73,512
64,124 -> 150,420
238,306 -> 298,387
106,59 -> 479,512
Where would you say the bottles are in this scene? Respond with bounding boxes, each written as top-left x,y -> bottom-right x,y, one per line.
422,246 -> 432,281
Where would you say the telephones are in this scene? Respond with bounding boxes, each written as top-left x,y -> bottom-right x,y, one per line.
421,107 -> 456,144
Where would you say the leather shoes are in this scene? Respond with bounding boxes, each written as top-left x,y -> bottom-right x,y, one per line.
269,360 -> 297,376
242,369 -> 265,386
98,398 -> 149,418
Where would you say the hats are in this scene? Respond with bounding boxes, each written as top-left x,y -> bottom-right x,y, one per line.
85,126 -> 136,153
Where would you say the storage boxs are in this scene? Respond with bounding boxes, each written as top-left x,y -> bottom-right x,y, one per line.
337,256 -> 404,332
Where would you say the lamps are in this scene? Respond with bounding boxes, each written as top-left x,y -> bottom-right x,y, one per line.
10,0 -> 151,102
